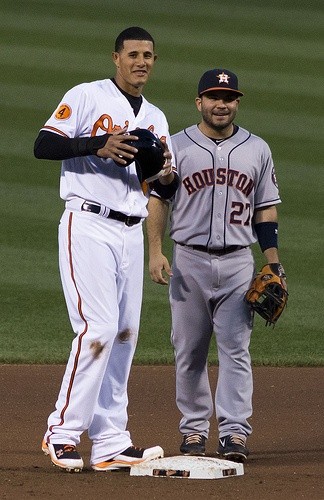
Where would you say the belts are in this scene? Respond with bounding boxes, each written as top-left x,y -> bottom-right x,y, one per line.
81,203 -> 141,227
174,240 -> 249,257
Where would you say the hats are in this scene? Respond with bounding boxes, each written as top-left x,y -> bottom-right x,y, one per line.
198,69 -> 244,98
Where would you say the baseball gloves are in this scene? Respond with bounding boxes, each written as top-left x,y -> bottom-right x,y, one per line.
245,263 -> 289,324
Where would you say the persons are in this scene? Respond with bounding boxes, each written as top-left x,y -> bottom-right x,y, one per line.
34,26 -> 180,473
145,69 -> 287,462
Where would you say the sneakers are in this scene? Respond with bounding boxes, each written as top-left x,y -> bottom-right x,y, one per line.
41,438 -> 84,472
180,434 -> 206,456
91,445 -> 164,472
216,434 -> 248,462
